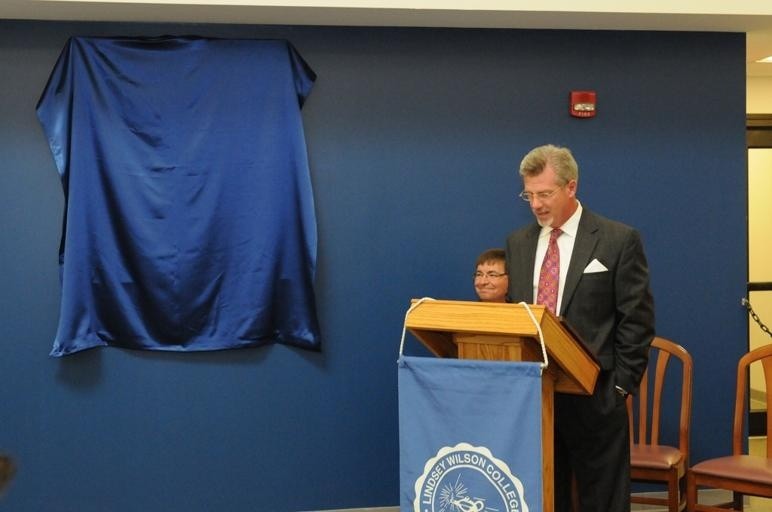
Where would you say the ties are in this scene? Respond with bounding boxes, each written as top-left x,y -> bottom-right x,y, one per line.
536,229 -> 565,318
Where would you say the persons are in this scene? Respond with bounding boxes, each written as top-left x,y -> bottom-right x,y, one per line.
506,144 -> 656,512
473,249 -> 512,302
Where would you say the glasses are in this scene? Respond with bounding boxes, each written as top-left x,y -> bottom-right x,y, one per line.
472,270 -> 507,280
518,184 -> 565,202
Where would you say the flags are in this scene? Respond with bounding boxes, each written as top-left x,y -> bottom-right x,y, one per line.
397,355 -> 544,512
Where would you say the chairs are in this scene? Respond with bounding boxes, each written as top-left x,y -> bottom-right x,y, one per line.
625,334 -> 693,511
684,342 -> 772,512
